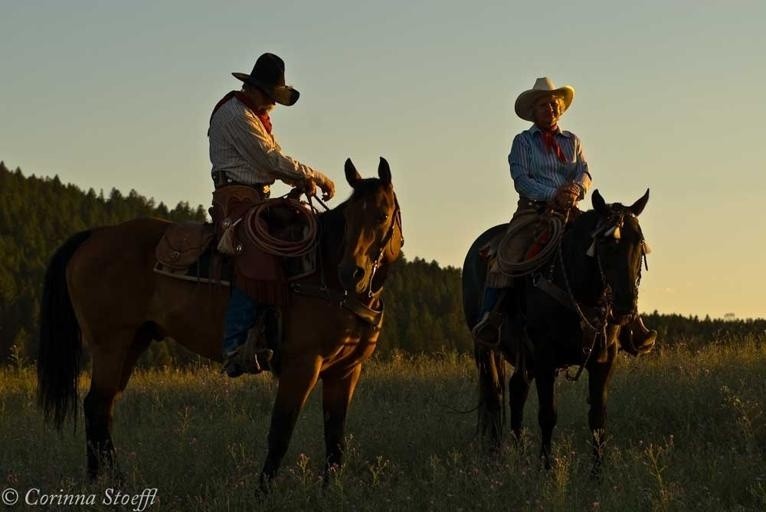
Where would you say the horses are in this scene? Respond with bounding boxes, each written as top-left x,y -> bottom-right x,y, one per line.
462,188 -> 650,474
35,156 -> 404,505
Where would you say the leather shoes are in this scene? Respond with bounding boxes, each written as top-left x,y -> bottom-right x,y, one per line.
225,345 -> 274,377
634,317 -> 656,350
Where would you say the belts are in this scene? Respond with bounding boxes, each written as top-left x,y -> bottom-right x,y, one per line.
215,176 -> 270,193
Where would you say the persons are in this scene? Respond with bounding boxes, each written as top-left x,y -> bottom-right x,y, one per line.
471,76 -> 659,362
205,50 -> 336,378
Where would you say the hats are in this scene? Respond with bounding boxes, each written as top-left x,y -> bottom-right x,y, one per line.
232,53 -> 299,106
515,77 -> 574,121
